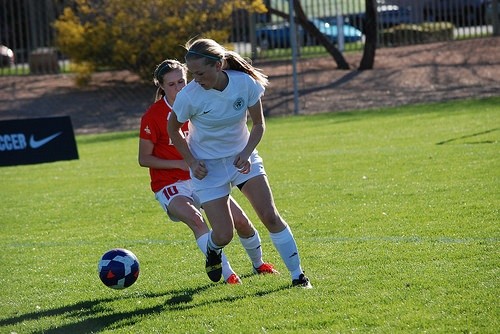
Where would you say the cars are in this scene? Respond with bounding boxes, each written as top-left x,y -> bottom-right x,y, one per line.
257,11 -> 362,51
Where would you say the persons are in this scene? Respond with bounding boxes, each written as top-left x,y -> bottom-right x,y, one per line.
167,38 -> 311,288
138,60 -> 278,282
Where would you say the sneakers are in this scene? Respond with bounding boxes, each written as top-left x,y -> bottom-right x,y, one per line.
291,272 -> 312,289
205,235 -> 224,281
252,262 -> 280,275
226,274 -> 242,283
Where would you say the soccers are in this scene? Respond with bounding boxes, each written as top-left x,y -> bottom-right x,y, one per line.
98,248 -> 140,289
